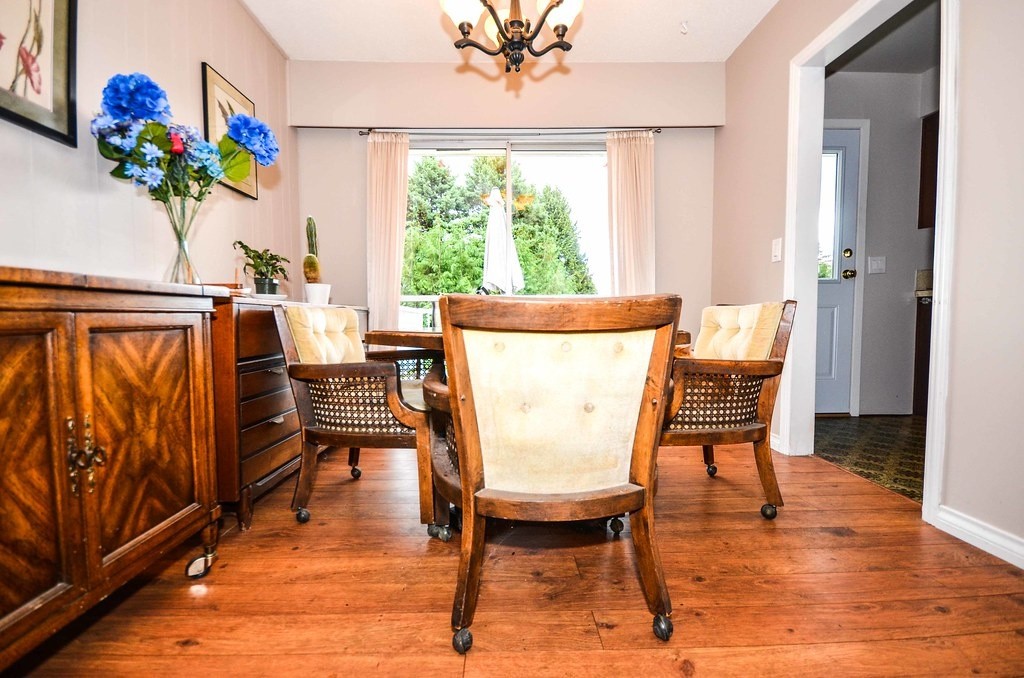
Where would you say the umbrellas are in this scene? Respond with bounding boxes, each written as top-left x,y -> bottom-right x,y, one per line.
482,186 -> 525,296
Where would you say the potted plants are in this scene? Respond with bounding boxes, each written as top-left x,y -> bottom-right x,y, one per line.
234,238 -> 290,294
303,215 -> 332,304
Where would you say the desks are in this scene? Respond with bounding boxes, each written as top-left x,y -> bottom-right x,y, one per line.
364,329 -> 691,349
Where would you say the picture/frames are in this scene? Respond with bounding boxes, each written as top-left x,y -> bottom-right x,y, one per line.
201,62 -> 258,200
0,0 -> 77,149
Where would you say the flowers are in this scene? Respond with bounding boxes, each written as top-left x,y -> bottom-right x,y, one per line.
89,74 -> 280,286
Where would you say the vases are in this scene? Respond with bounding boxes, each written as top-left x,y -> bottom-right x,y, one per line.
161,241 -> 203,285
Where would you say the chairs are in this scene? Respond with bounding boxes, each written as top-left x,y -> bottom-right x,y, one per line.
660,299 -> 798,519
271,304 -> 440,535
423,291 -> 683,654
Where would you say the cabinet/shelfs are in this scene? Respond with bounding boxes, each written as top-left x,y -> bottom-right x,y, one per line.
0,260 -> 231,667
208,299 -> 303,532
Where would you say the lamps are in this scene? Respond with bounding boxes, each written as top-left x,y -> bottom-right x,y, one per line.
438,0 -> 585,74
